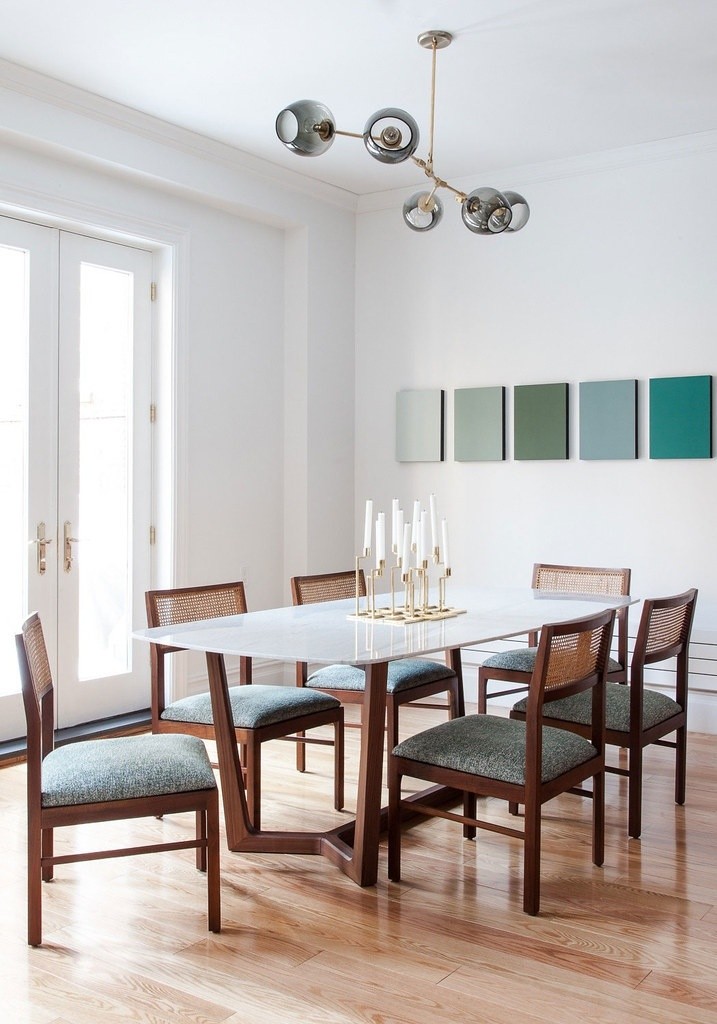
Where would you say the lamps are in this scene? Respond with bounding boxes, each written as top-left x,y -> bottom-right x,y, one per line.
275,30 -> 530,233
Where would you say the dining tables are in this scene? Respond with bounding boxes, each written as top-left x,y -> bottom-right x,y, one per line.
128,588 -> 641,887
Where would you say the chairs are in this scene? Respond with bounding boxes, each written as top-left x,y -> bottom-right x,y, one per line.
290,569 -> 461,788
15,610 -> 221,948
506,587 -> 699,839
145,582 -> 345,833
476,562 -> 632,715
389,608 -> 618,915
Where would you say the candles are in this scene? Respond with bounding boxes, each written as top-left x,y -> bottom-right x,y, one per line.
363,490 -> 451,583
